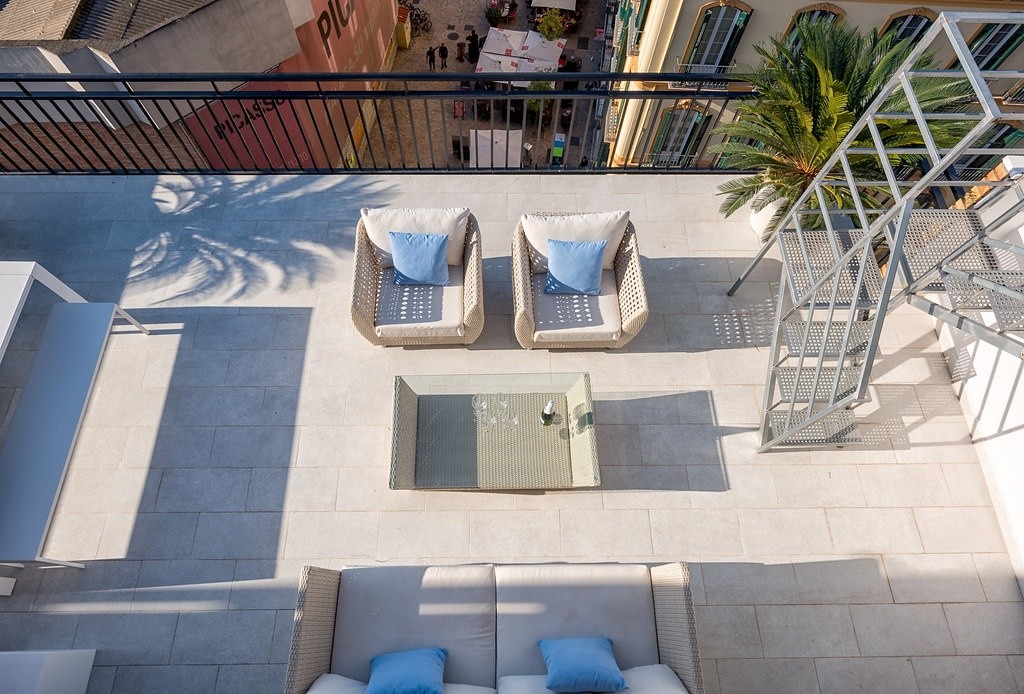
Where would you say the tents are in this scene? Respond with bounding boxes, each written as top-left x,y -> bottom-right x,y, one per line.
475,26 -> 567,89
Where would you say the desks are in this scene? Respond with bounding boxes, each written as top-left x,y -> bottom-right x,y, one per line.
388,371 -> 602,491
0,260 -> 89,596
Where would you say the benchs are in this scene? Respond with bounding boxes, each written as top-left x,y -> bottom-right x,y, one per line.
283,560 -> 705,694
0,303 -> 150,569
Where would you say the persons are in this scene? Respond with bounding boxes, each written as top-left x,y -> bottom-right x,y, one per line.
426,46 -> 439,72
553,157 -> 560,165
581,156 -> 588,167
438,43 -> 448,69
585,80 -> 594,91
509,0 -> 518,12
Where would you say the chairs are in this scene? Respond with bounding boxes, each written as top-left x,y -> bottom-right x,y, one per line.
507,7 -> 519,25
511,212 -> 650,351
348,213 -> 484,348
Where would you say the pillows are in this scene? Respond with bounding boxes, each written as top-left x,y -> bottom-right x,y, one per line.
537,636 -> 630,694
520,210 -> 630,274
389,231 -> 453,287
360,207 -> 470,269
365,647 -> 448,694
542,239 -> 608,296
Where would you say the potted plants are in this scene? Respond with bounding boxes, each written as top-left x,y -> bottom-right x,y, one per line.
484,8 -> 502,28
704,13 -> 1001,243
